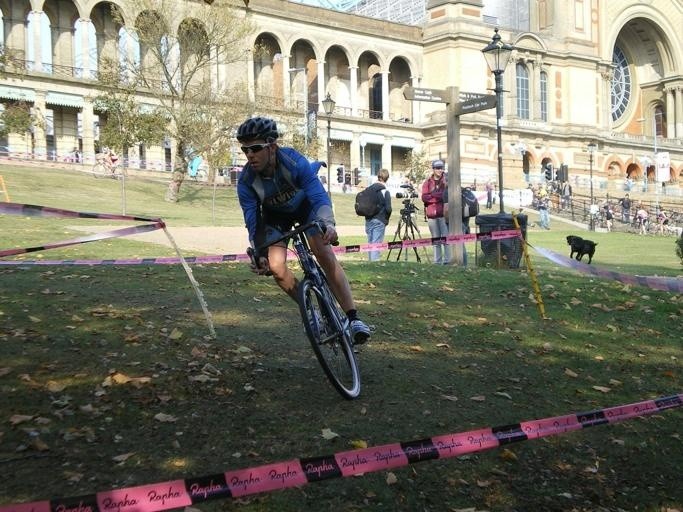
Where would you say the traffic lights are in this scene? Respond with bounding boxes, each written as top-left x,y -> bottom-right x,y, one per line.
555,170 -> 561,181
545,163 -> 554,182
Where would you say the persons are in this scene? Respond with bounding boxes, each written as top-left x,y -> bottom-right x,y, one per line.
441,169 -> 470,267
71,146 -> 80,163
362,168 -> 392,260
526,179 -> 674,237
485,178 -> 495,210
97,146 -> 120,175
420,159 -> 453,268
232,115 -> 368,347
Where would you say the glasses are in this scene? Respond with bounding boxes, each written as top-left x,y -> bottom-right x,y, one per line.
240,144 -> 270,152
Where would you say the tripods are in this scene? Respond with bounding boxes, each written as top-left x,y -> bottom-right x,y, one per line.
387,199 -> 432,263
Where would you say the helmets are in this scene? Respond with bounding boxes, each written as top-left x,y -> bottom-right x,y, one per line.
237,117 -> 278,140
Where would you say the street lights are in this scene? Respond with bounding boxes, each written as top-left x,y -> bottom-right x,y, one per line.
322,92 -> 336,200
587,143 -> 596,231
475,28 -> 528,270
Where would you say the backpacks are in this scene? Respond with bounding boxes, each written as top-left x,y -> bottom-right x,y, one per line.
462,187 -> 479,218
355,185 -> 385,216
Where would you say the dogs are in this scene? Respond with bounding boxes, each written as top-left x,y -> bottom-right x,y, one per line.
565,234 -> 597,264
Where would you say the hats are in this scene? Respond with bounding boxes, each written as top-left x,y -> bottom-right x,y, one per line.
432,160 -> 444,168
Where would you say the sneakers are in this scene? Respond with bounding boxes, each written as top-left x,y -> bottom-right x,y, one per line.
349,320 -> 370,342
303,309 -> 320,333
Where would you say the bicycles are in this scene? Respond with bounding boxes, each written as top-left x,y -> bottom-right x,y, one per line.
247,218 -> 367,400
93,153 -> 126,180
